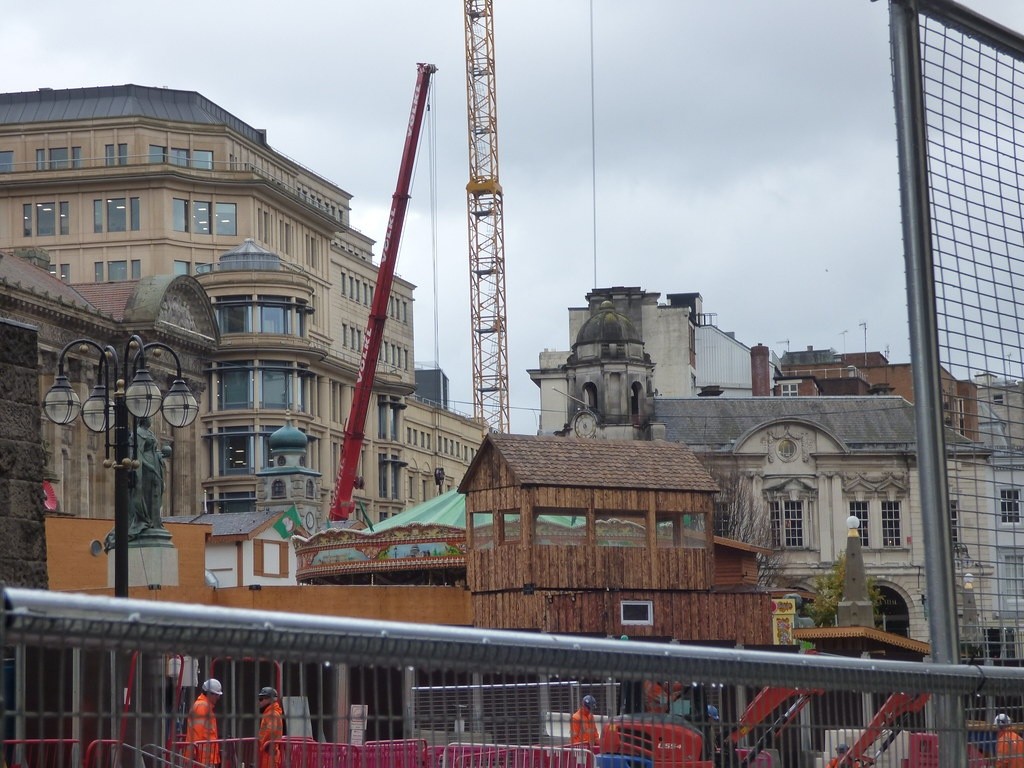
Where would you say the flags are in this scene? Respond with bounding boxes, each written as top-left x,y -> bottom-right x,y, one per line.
360,502 -> 375,532
326,518 -> 331,529
273,505 -> 302,539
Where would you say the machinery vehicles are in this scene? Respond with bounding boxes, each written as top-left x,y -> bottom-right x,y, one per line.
598,648 -> 929,768
327,60 -> 446,526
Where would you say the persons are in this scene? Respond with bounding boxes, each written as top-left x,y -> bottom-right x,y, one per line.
708,705 -> 719,721
258,687 -> 282,768
570,696 -> 599,743
104,417 -> 172,555
644,680 -> 681,714
184,679 -> 223,768
993,714 -> 1024,768
826,744 -> 859,768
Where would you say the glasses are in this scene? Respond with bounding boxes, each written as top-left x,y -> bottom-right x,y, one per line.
259,696 -> 269,702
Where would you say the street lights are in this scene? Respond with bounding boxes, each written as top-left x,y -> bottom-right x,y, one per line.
40,332 -> 203,599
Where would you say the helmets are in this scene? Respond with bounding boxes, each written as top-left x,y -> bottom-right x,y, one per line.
258,687 -> 278,697
202,678 -> 222,696
836,743 -> 848,752
705,705 -> 719,721
993,713 -> 1011,725
583,695 -> 596,707
